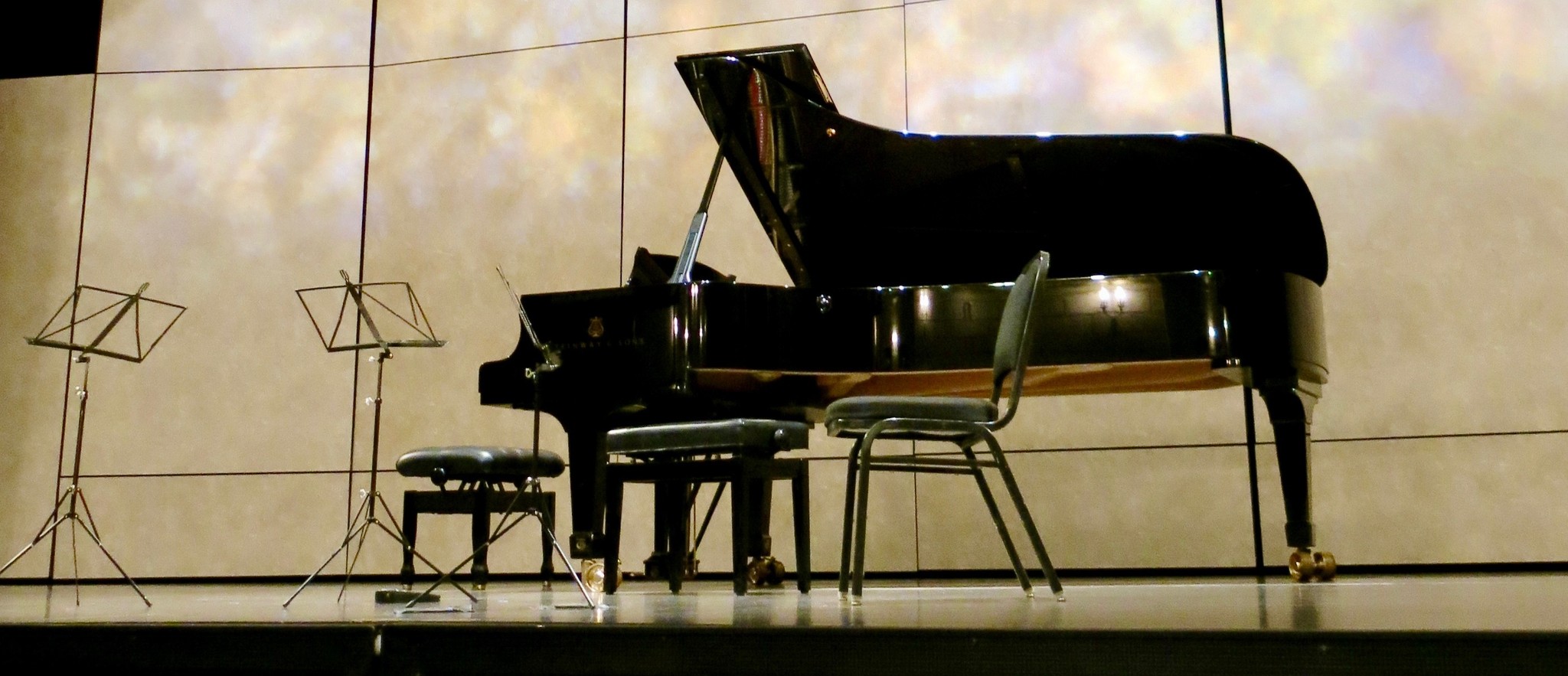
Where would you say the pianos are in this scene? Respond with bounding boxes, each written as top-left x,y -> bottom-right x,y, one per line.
477,42 -> 1337,596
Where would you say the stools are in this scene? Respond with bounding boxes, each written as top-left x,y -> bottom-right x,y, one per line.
396,447 -> 565,591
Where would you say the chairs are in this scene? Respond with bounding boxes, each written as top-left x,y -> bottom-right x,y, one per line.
822,249 -> 1067,604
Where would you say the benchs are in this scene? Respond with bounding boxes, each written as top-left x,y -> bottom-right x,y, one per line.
605,417 -> 812,597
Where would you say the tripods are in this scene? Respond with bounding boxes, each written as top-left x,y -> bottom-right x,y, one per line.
281,265 -> 596,615
1,282 -> 187,608
